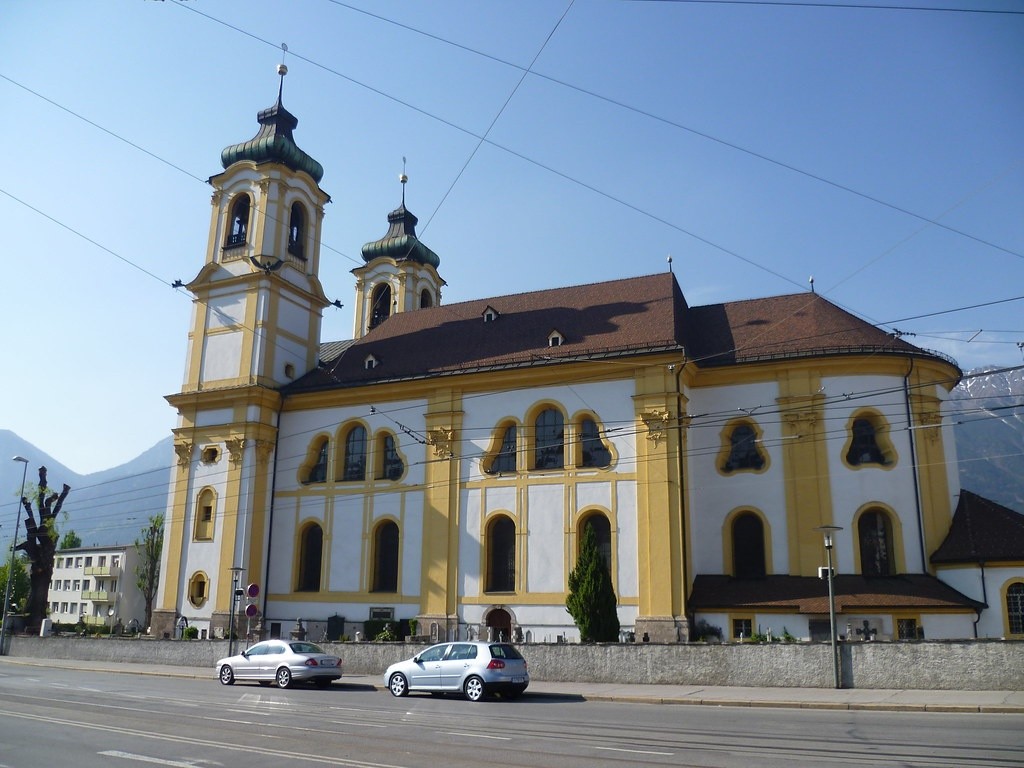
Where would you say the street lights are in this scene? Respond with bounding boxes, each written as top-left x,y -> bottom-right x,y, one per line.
0,452 -> 30,658
225,566 -> 247,657
811,523 -> 845,689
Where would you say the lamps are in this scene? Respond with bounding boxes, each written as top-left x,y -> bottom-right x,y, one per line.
331,299 -> 344,308
172,280 -> 186,288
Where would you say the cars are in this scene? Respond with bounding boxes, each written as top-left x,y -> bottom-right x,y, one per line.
217,637 -> 343,689
383,641 -> 529,702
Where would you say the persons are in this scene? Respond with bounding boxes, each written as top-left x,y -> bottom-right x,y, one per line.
433,649 -> 443,661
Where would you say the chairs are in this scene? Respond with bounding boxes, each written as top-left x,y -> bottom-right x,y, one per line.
294,644 -> 302,651
